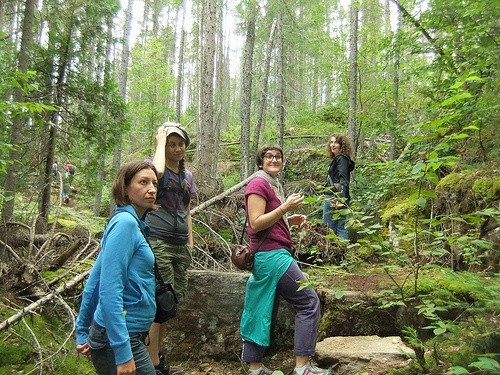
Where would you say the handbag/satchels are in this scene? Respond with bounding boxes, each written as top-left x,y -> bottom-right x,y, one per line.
151,281 -> 180,325
231,245 -> 254,270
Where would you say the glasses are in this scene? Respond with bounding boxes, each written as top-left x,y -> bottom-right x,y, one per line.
263,154 -> 282,161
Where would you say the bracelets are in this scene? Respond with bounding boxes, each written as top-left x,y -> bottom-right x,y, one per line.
280,207 -> 285,214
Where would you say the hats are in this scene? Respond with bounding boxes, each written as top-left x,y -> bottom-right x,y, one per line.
161,122 -> 191,148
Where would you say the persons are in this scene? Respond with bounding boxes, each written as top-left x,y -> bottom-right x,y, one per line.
322,134 -> 355,238
75,160 -> 159,375
62,162 -> 74,203
240,145 -> 331,375
52,161 -> 57,175
146,121 -> 196,375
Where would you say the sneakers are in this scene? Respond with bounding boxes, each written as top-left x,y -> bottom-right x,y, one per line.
293,360 -> 331,375
154,362 -> 170,375
247,362 -> 274,375
